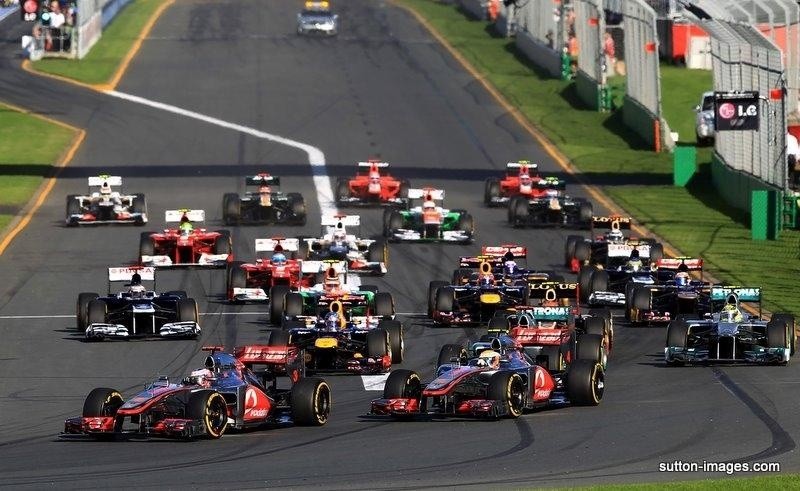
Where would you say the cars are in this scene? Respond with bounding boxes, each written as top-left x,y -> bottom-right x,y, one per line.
57,158 -> 794,443
694,90 -> 719,147
296,2 -> 337,38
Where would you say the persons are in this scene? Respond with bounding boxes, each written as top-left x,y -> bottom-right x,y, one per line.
48,7 -> 66,50
